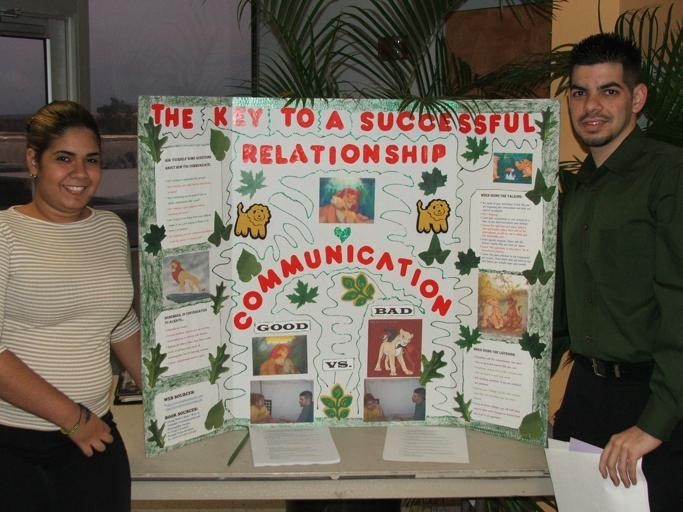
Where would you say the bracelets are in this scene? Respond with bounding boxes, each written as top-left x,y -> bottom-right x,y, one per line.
60,404 -> 92,435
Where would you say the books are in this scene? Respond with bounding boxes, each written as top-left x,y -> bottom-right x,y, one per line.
381,425 -> 470,464
545,438 -> 652,512
248,424 -> 342,466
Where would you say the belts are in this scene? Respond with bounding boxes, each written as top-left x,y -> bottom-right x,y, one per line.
568,351 -> 653,380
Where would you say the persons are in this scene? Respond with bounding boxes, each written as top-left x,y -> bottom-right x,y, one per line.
0,100 -> 142,512
250,394 -> 274,422
549,33 -> 683,512
284,391 -> 313,422
411,388 -> 425,420
